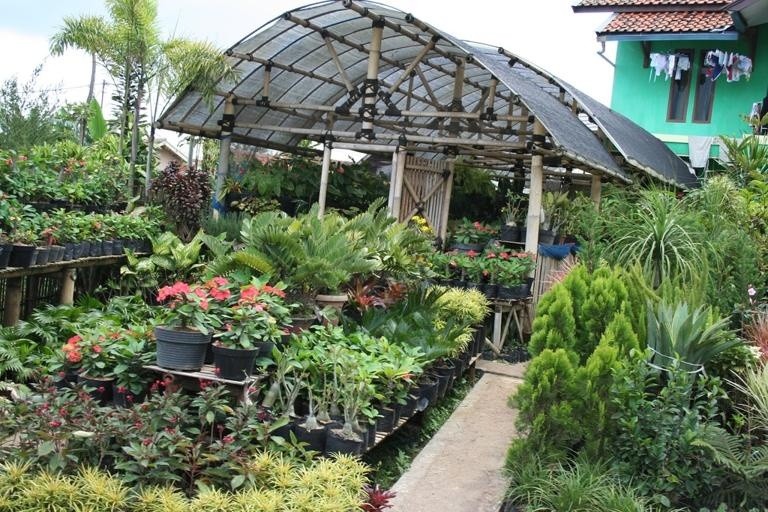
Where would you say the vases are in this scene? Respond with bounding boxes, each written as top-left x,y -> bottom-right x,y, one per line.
0,239 -> 148,268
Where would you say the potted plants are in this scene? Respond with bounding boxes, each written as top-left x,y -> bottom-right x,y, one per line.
208,190 -> 594,458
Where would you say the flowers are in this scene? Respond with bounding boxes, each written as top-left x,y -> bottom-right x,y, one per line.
0,141 -> 151,239
0,275 -> 290,497
